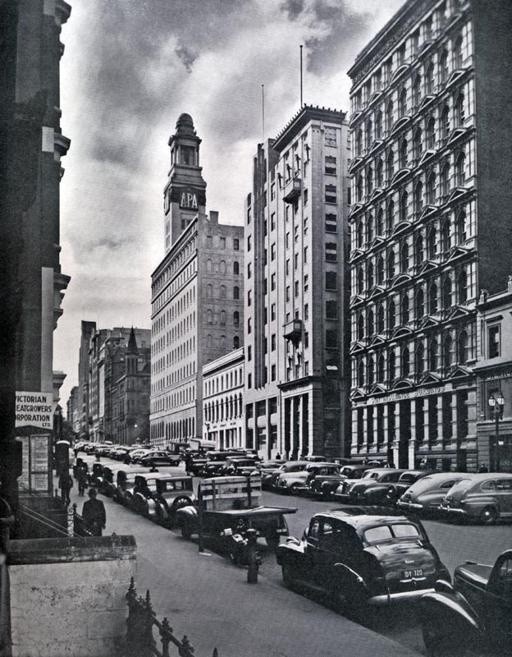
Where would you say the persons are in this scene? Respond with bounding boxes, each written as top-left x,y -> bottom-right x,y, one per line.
478,464 -> 488,473
59,469 -> 73,505
82,489 -> 106,536
75,466 -> 87,496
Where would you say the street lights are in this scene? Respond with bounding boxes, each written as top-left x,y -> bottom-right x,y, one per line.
487,393 -> 506,469
